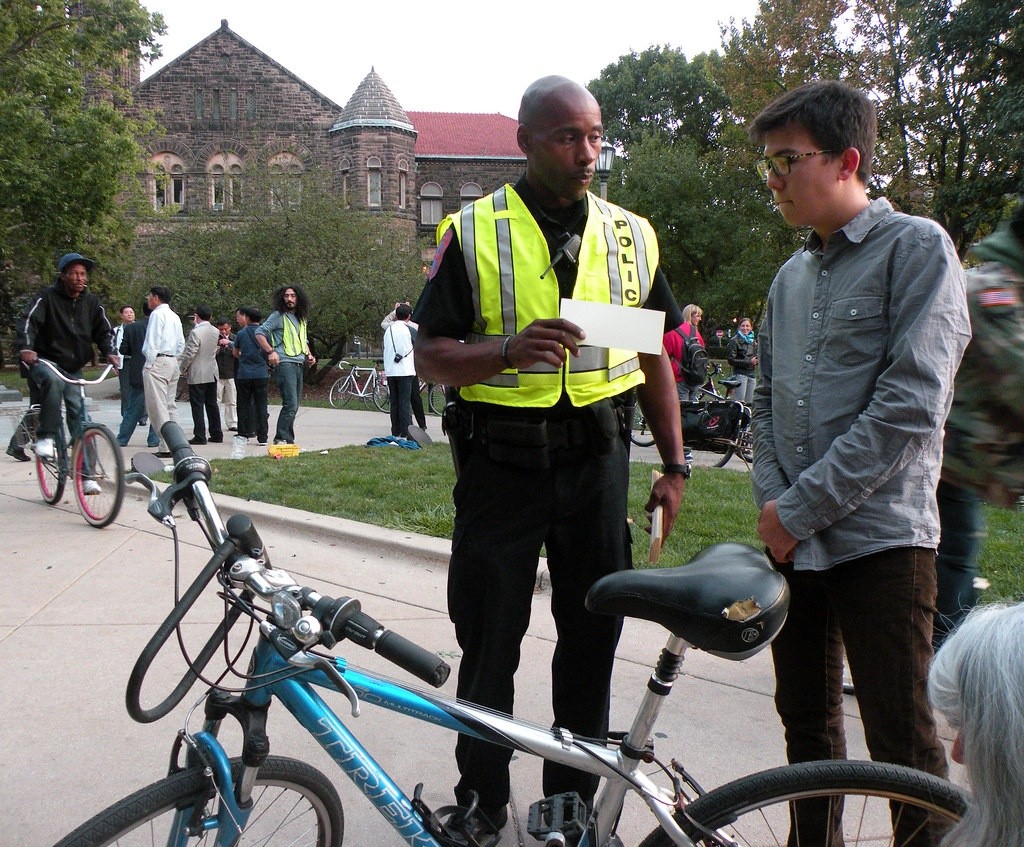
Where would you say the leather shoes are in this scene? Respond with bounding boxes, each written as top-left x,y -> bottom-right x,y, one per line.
151,452 -> 172,458
5,446 -> 31,461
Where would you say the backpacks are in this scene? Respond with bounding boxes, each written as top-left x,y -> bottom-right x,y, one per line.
674,325 -> 708,386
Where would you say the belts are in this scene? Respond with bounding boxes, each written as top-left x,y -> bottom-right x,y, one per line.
157,353 -> 172,357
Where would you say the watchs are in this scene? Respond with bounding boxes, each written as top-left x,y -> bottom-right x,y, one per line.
661,463 -> 691,478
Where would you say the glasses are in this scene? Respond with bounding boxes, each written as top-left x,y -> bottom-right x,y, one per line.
754,149 -> 833,181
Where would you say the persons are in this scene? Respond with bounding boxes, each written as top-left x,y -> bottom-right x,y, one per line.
380,302 -> 427,440
255,285 -> 316,445
925,602 -> 1024,847
179,304 -> 224,444
110,302 -> 160,447
930,196 -> 1023,649
409,75 -> 683,847
215,319 -> 238,430
5,253 -> 121,494
727,318 -> 758,429
140,286 -> 185,458
664,303 -> 705,463
219,307 -> 271,445
741,78 -> 970,847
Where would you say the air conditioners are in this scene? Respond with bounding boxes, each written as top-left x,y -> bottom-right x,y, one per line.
213,202 -> 224,211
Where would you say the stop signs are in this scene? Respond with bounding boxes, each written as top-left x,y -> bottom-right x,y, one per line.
715,330 -> 725,338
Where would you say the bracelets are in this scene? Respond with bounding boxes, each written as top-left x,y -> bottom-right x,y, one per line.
502,335 -> 513,368
268,349 -> 274,354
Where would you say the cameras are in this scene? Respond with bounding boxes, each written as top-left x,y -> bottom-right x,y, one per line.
220,344 -> 225,353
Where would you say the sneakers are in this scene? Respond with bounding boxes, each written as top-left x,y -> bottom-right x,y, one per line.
82,480 -> 102,496
34,438 -> 54,459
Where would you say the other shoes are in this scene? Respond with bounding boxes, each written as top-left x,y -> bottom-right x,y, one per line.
562,811 -> 625,847
229,427 -> 237,431
208,437 -> 224,443
188,437 -> 207,445
259,440 -> 268,446
139,421 -> 146,425
441,804 -> 508,840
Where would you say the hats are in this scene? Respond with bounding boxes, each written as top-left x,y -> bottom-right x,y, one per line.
974,204 -> 1023,270
58,253 -> 94,273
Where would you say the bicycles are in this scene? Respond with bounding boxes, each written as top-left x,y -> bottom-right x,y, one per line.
622,360 -> 754,468
9,353 -> 134,529
328,358 -> 389,410
53,420 -> 973,847
372,372 -> 446,417
